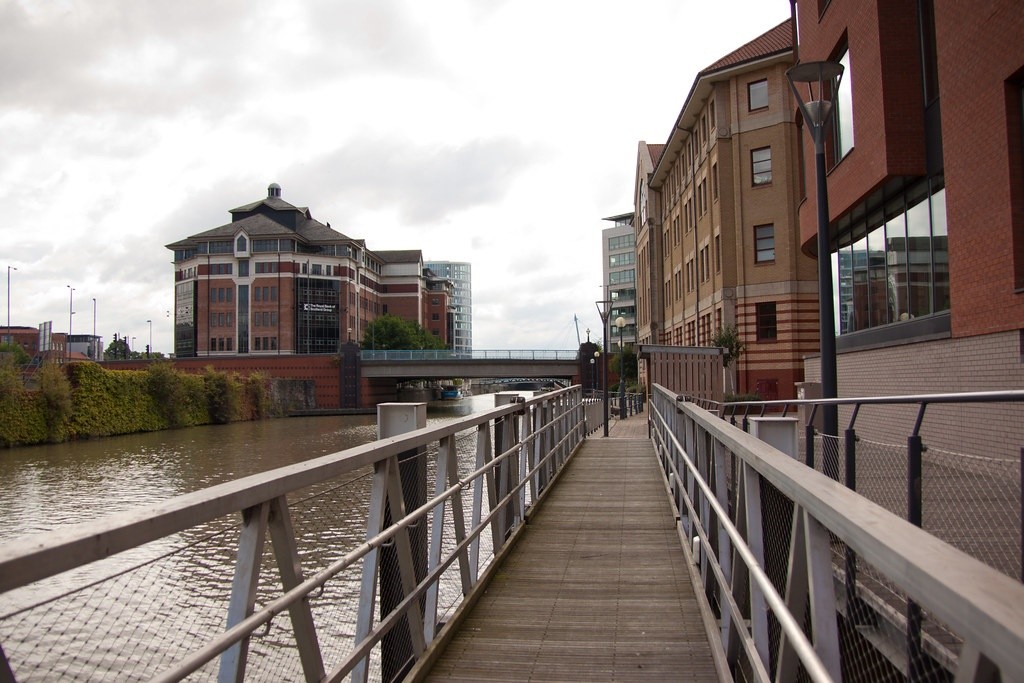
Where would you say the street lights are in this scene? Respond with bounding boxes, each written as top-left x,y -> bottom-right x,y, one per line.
7,266 -> 18,353
590,359 -> 595,398
66,285 -> 76,362
786,58 -> 845,549
594,351 -> 599,394
596,300 -> 614,438
615,316 -> 626,421
147,320 -> 152,359
92,298 -> 97,361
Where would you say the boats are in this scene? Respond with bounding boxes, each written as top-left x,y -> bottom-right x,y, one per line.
440,388 -> 464,401
